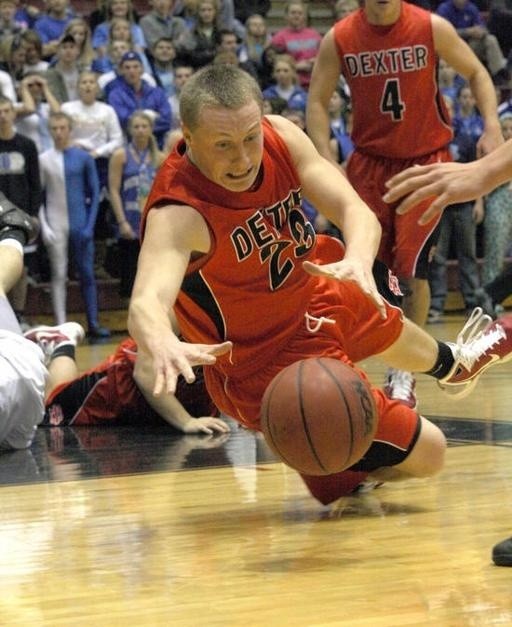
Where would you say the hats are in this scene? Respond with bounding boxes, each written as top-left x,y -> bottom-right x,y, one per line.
122,51 -> 141,63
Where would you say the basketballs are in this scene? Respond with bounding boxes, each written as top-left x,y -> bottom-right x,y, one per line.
262,358 -> 378,477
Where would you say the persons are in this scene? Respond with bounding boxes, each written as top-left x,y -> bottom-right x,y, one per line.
0,0 -> 512,410
124,63 -> 511,510
23,320 -> 235,438
0,190 -> 50,455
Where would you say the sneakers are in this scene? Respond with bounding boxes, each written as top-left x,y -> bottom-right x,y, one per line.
438,313 -> 512,399
492,537 -> 512,566
22,322 -> 86,355
0,191 -> 39,246
88,327 -> 110,337
427,308 -> 440,323
383,367 -> 418,411
